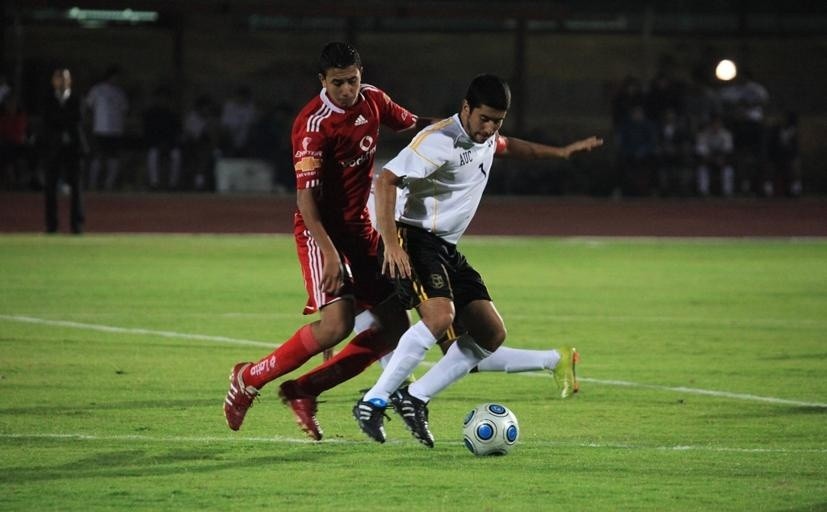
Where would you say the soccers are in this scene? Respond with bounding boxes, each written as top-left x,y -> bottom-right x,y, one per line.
463,403 -> 518,455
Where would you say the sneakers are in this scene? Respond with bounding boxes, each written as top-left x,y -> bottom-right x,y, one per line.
550,346 -> 579,399
223,362 -> 260,431
352,373 -> 435,449
278,379 -> 326,441
